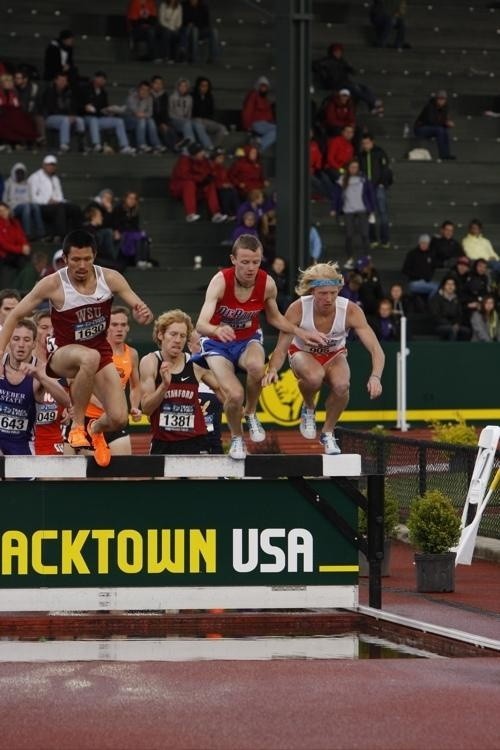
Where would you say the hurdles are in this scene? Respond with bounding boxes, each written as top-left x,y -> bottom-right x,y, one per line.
0,454 -> 384,610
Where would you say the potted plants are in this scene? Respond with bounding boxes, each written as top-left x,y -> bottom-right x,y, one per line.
360,423 -> 396,475
426,410 -> 480,475
359,478 -> 402,578
404,490 -> 459,593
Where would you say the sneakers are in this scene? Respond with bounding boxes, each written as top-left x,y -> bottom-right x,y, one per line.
67,423 -> 91,449
212,212 -> 228,223
1,137 -> 192,157
319,430 -> 342,455
87,418 -> 112,468
186,212 -> 200,223
228,432 -> 248,460
299,402 -> 317,441
244,411 -> 267,443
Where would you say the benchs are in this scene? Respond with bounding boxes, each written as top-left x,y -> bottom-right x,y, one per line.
1,1 -> 499,347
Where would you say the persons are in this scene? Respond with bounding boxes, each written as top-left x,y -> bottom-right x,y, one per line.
0,227 -> 155,468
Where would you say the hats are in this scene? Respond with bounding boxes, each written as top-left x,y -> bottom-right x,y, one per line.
418,233 -> 430,244
187,141 -> 205,157
458,257 -> 470,267
43,155 -> 59,164
